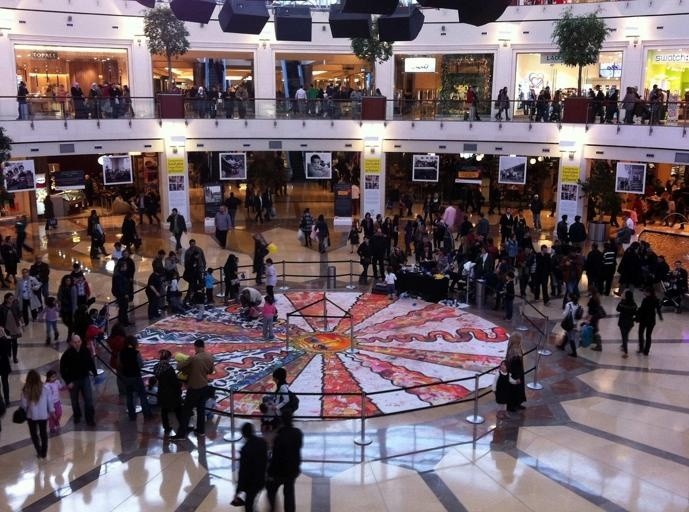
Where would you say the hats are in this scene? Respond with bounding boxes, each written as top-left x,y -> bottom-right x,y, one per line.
504,271 -> 515,279
501,256 -> 508,260
575,215 -> 581,221
73,260 -> 81,268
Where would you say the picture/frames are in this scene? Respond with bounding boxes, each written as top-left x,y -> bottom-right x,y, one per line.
614,161 -> 647,194
3,159 -> 36,193
497,155 -> 527,184
411,155 -> 440,183
102,155 -> 134,186
305,151 -> 333,180
218,153 -> 248,182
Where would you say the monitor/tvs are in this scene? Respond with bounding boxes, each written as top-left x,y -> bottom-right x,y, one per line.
404,57 -> 436,73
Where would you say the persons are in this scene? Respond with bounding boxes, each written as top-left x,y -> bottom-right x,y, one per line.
231,423 -> 269,511
266,418 -> 304,512
1,71 -> 688,440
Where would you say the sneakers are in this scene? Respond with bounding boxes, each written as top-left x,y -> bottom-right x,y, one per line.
507,405 -> 526,412
13,356 -> 18,363
74,414 -> 82,423
6,399 -> 10,407
130,412 -> 137,420
162,422 -> 206,444
614,291 -> 622,296
22,317 -> 59,347
496,410 -> 509,420
590,118 -> 663,125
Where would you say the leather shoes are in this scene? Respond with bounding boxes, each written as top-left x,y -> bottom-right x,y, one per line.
120,320 -> 135,327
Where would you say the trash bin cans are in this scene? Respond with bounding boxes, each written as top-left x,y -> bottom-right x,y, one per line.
475,278 -> 486,308
328,266 -> 336,289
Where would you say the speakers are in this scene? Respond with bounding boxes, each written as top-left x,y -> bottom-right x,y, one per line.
170,0 -> 217,23
275,7 -> 312,42
458,0 -> 513,26
218,1 -> 269,34
340,1 -> 400,16
417,0 -> 457,9
138,0 -> 155,9
377,6 -> 425,41
329,4 -> 372,38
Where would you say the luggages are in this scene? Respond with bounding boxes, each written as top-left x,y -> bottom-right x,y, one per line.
581,324 -> 592,348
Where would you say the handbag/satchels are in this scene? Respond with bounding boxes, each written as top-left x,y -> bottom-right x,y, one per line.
280,393 -> 299,414
270,207 -> 276,217
48,218 -> 57,226
328,238 -> 330,245
561,308 -> 574,331
120,236 -> 141,250
12,408 -> 27,423
519,266 -> 529,277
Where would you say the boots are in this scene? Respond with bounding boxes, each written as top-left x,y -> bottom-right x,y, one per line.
555,340 -> 578,358
591,335 -> 602,351
